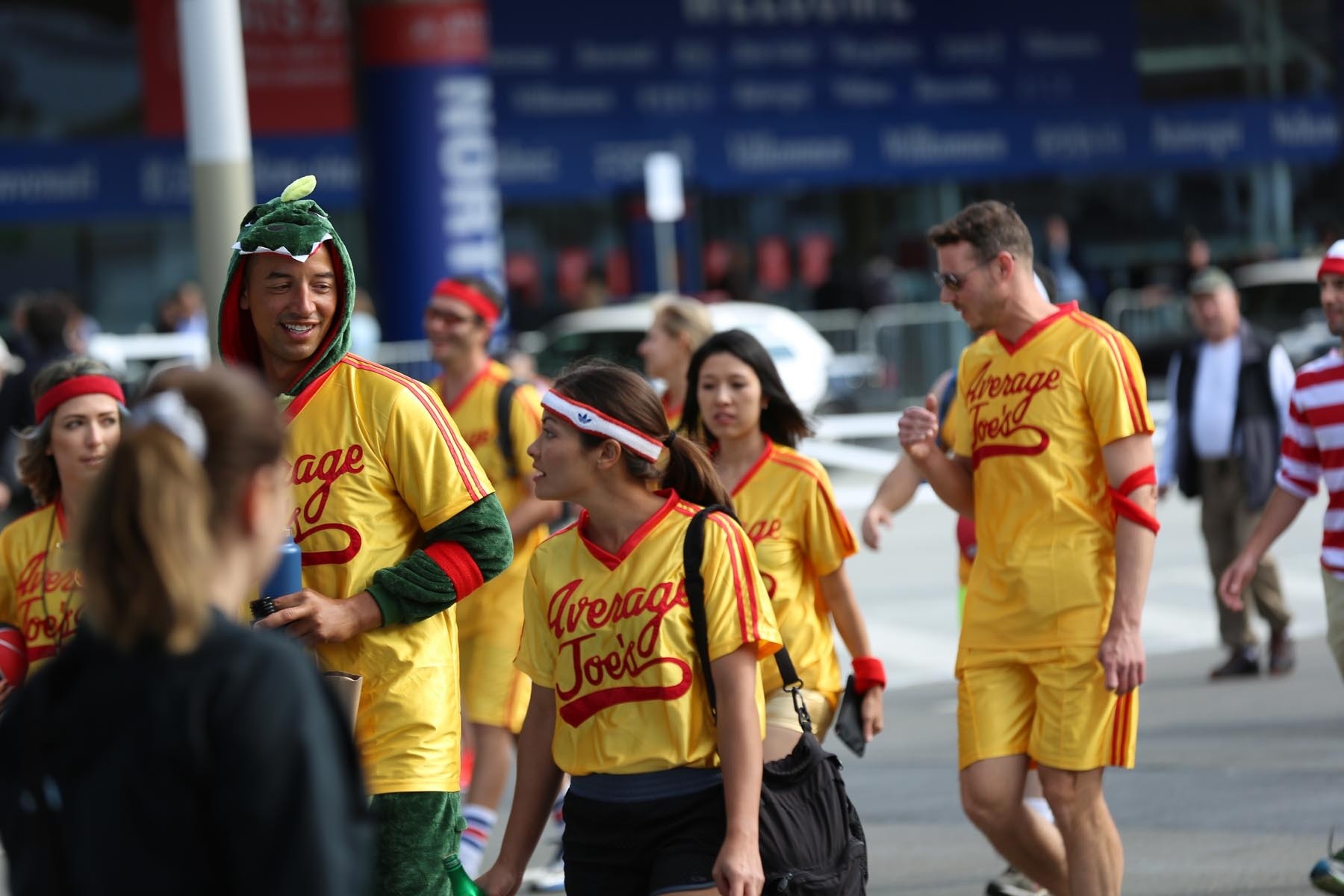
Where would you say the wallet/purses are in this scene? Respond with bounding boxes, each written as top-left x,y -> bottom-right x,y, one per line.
834,675 -> 870,758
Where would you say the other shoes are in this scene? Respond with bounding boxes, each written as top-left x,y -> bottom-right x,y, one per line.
522,837 -> 566,892
1270,637 -> 1297,676
1211,652 -> 1259,677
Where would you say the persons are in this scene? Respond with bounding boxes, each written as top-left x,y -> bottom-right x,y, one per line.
1033,214 -> 1210,310
1218,240 -> 1344,896
471,365 -> 781,896
425,277 -> 570,890
0,357 -> 132,712
217,174 -> 514,896
0,281 -> 209,511
897,201 -> 1159,896
864,265 -> 1296,678
638,298 -> 885,761
0,366 -> 372,896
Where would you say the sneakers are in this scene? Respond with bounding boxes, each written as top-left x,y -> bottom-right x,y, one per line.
986,868 -> 1049,896
1310,828 -> 1343,896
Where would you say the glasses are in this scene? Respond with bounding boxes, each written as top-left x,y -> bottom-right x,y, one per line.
933,254 -> 995,292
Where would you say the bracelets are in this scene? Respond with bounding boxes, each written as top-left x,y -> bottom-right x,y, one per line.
851,658 -> 885,692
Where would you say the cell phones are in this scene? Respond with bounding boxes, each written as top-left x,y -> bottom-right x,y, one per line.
835,674 -> 865,758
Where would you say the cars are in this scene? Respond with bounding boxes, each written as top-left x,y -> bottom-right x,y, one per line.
539,297 -> 830,429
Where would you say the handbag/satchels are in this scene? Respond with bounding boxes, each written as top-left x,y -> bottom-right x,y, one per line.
725,728 -> 870,896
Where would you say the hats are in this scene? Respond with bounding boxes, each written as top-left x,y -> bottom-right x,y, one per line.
1186,265 -> 1234,293
1317,238 -> 1344,283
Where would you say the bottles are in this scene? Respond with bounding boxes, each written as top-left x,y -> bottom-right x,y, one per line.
254,529 -> 305,611
